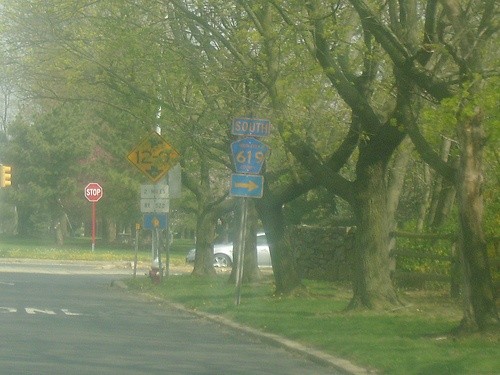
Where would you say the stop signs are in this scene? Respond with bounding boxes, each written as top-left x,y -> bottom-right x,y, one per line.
84,182 -> 103,203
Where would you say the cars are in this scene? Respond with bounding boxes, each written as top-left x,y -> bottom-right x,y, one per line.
185,232 -> 271,268
